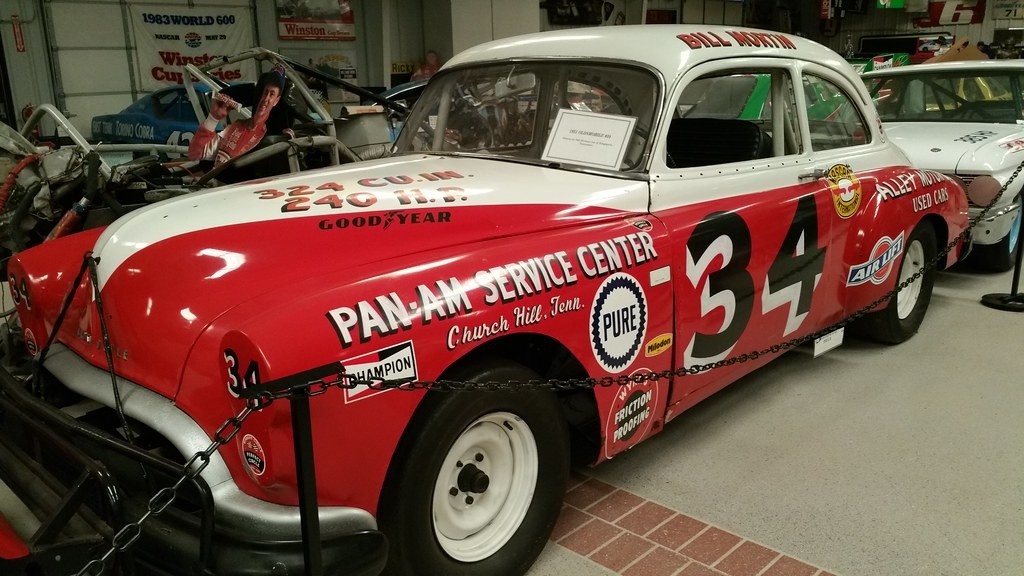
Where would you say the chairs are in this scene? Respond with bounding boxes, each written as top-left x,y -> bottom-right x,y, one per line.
666,118 -> 773,168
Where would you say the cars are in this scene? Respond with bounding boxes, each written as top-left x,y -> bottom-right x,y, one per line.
795,59 -> 1024,272
0,25 -> 970,576
91,82 -> 424,173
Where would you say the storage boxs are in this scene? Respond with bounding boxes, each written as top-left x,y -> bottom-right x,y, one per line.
337,111 -> 393,160
